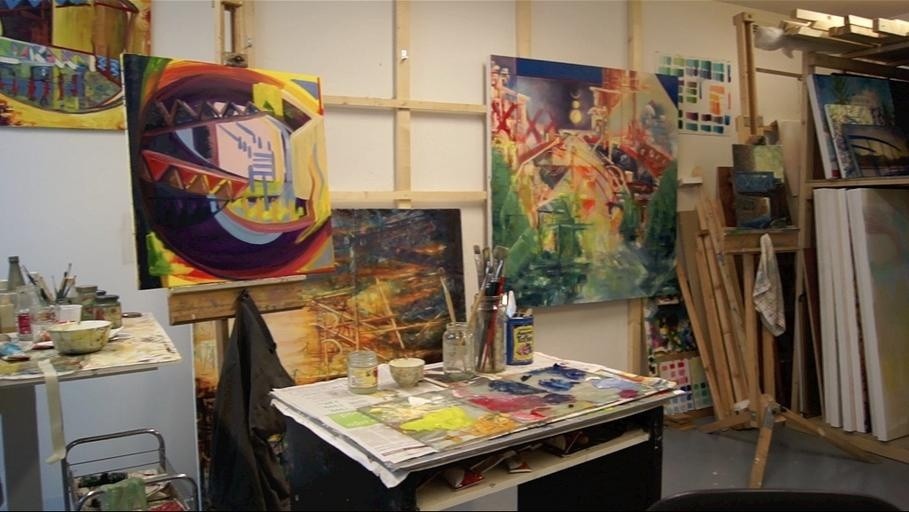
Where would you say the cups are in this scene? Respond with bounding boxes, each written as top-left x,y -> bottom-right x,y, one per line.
504,313 -> 534,367
53,304 -> 83,324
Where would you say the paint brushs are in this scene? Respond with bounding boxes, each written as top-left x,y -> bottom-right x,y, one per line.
22,262 -> 77,306
439,245 -> 509,375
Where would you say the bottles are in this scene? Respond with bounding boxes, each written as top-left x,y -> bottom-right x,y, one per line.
7,255 -> 26,306
468,293 -> 508,374
442,322 -> 477,381
27,272 -> 43,322
13,285 -> 36,342
74,285 -> 123,330
345,350 -> 379,395
39,297 -> 71,321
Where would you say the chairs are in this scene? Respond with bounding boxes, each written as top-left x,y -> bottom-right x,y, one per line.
651,489 -> 906,512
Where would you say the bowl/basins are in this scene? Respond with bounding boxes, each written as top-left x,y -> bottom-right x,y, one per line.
389,357 -> 424,390
45,318 -> 112,356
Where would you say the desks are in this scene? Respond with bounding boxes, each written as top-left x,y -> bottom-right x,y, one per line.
1,312 -> 160,511
270,347 -> 690,512
